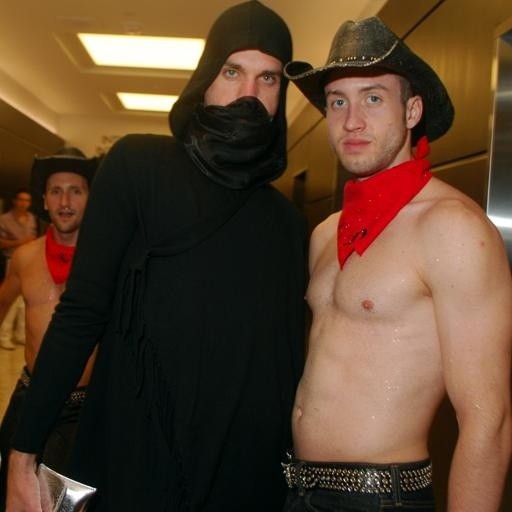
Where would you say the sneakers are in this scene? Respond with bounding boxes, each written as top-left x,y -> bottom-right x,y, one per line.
0,337 -> 26,350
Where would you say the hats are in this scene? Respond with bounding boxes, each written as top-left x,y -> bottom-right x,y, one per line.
30,145 -> 103,225
284,16 -> 454,145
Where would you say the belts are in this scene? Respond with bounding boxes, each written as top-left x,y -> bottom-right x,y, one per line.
18,366 -> 90,405
281,459 -> 437,496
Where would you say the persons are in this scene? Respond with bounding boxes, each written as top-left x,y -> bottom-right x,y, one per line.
270,9 -> 512,509
2,2 -> 312,512
1,146 -> 103,476
0,190 -> 42,350
